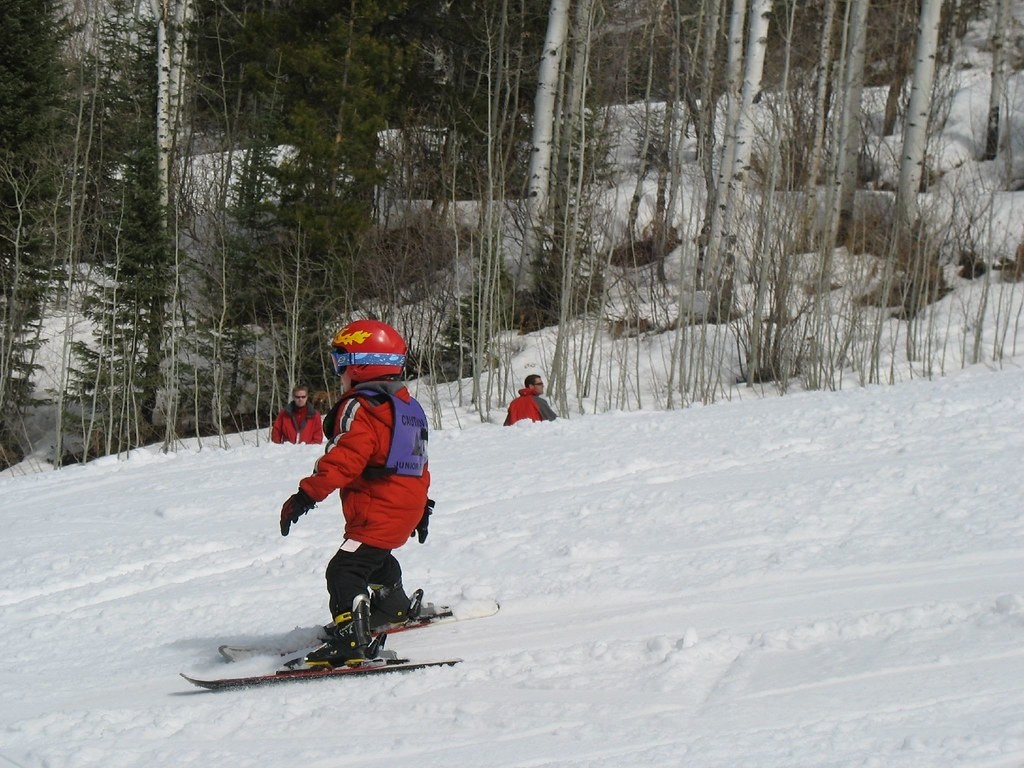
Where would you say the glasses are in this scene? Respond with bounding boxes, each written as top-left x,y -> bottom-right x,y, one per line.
330,352 -> 406,375
535,383 -> 543,385
295,396 -> 306,398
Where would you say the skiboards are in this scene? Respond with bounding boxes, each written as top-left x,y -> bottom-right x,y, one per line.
179,598 -> 501,689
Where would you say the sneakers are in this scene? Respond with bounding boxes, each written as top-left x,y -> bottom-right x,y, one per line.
304,593 -> 372,668
368,576 -> 411,630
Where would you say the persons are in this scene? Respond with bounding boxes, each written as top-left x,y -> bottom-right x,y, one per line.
280,319 -> 435,668
504,374 -> 558,427
270,386 -> 323,445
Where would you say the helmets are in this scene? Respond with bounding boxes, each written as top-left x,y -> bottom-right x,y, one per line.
333,320 -> 407,382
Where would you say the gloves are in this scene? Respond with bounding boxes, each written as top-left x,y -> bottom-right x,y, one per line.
280,487 -> 316,536
411,499 -> 435,544
